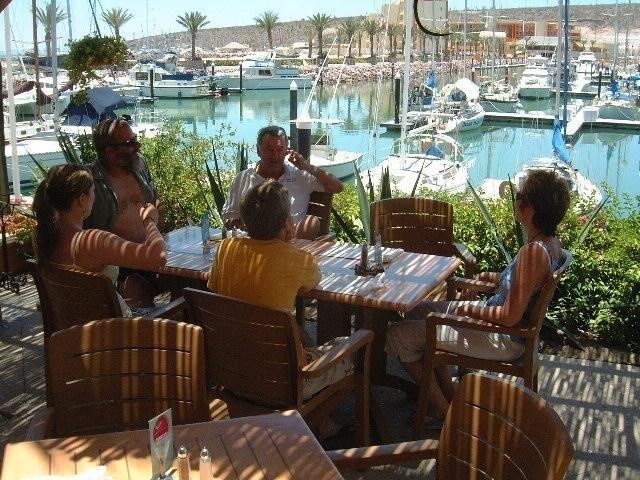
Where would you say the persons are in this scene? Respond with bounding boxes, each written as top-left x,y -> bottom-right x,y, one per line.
385,169 -> 570,430
32,164 -> 191,325
207,181 -> 356,442
221,126 -> 344,241
81,118 -> 206,311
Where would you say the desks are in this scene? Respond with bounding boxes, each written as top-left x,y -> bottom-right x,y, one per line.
0,409 -> 348,480
122,225 -> 461,392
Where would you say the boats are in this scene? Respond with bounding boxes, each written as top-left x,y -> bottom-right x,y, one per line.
246,35 -> 363,181
107,60 -> 212,100
215,50 -> 312,92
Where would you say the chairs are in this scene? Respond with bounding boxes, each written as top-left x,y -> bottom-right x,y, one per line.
31,265 -> 187,407
324,371 -> 575,480
26,317 -> 207,442
183,288 -> 375,447
412,248 -> 572,442
369,196 -> 477,280
305,192 -> 338,242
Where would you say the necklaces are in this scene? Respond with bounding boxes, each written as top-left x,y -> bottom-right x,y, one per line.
107,169 -> 129,181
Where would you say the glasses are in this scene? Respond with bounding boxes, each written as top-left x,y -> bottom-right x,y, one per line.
113,140 -> 135,148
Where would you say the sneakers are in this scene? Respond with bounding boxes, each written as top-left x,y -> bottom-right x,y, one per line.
319,416 -> 341,441
410,415 -> 444,430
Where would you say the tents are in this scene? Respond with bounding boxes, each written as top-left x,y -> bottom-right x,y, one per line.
60,86 -> 127,128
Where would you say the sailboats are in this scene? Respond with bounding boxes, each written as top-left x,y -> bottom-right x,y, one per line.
2,0 -> 157,192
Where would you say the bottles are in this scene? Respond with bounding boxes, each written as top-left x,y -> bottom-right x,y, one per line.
176,444 -> 191,480
200,210 -> 211,245
373,232 -> 384,265
198,445 -> 213,480
221,225 -> 227,239
361,239 -> 369,268
231,226 -> 238,238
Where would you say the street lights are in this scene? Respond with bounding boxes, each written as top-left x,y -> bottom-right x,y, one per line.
296,108 -> 312,167
239,93 -> 244,126
204,60 -> 207,72
289,80 -> 298,149
211,62 -> 215,76
239,60 -> 242,91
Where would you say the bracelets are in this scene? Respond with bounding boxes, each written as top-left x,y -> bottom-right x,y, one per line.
310,167 -> 318,176
143,217 -> 155,226
